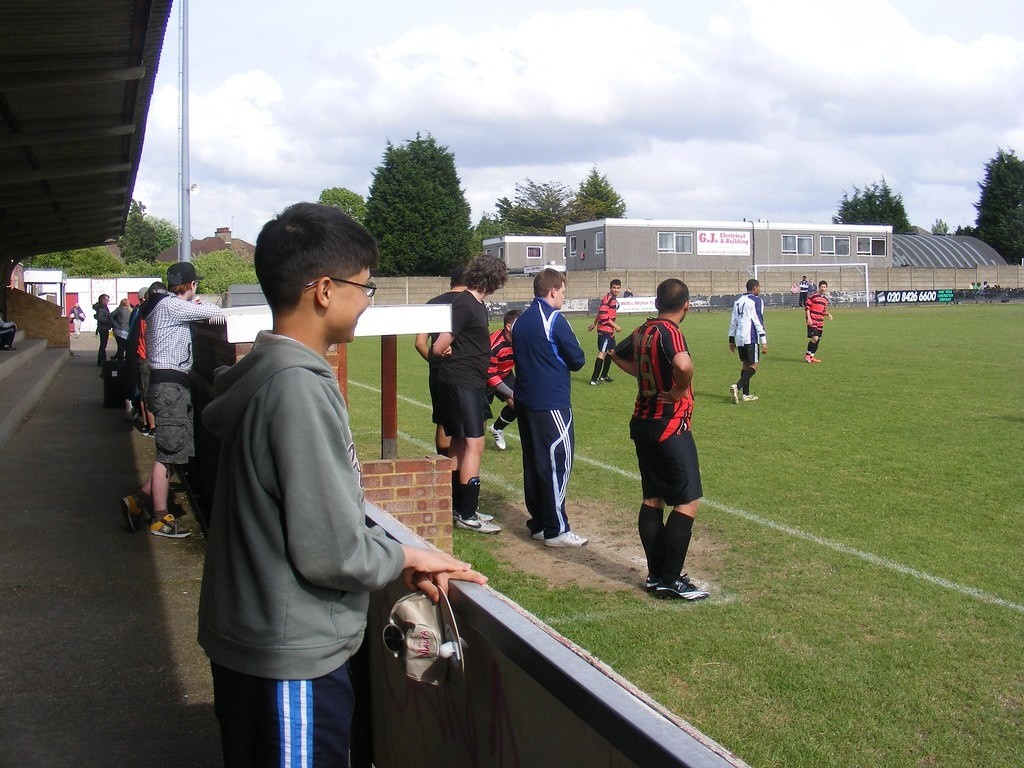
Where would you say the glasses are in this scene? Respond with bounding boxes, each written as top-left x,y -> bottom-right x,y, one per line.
305,276 -> 376,297
383,619 -> 404,658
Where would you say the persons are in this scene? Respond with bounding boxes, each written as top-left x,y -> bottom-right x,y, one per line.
799,276 -> 817,307
69,303 -> 86,337
197,202 -> 489,768
624,287 -> 633,298
485,309 -> 517,449
0,313 -> 17,351
127,282 -> 167,438
510,268 -> 588,548
968,281 -> 988,289
122,261 -> 221,537
804,281 -> 833,362
109,299 -> 133,360
587,279 -> 622,386
611,278 -> 710,601
433,253 -> 508,534
92,294 -> 110,366
415,267 -> 467,457
728,279 -> 768,403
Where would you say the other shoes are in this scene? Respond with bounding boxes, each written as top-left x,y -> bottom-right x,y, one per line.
810,357 -> 822,362
97,353 -> 158,438
598,377 -> 615,382
589,379 -> 601,385
804,354 -> 811,363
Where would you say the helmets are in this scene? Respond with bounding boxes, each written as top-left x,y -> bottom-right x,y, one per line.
149,282 -> 167,297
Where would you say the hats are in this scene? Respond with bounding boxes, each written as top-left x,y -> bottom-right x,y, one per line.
166,262 -> 205,287
382,580 -> 468,708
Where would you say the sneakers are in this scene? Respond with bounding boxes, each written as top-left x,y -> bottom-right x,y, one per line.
743,394 -> 758,402
453,509 -> 494,522
544,530 -> 589,548
486,423 -> 507,450
456,512 -> 502,534
730,384 -> 739,404
145,512 -> 191,539
532,531 -> 545,540
121,496 -> 143,532
646,575 -> 701,593
656,574 -> 711,601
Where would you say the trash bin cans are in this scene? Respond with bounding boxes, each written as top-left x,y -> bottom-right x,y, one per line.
103,361 -> 128,408
